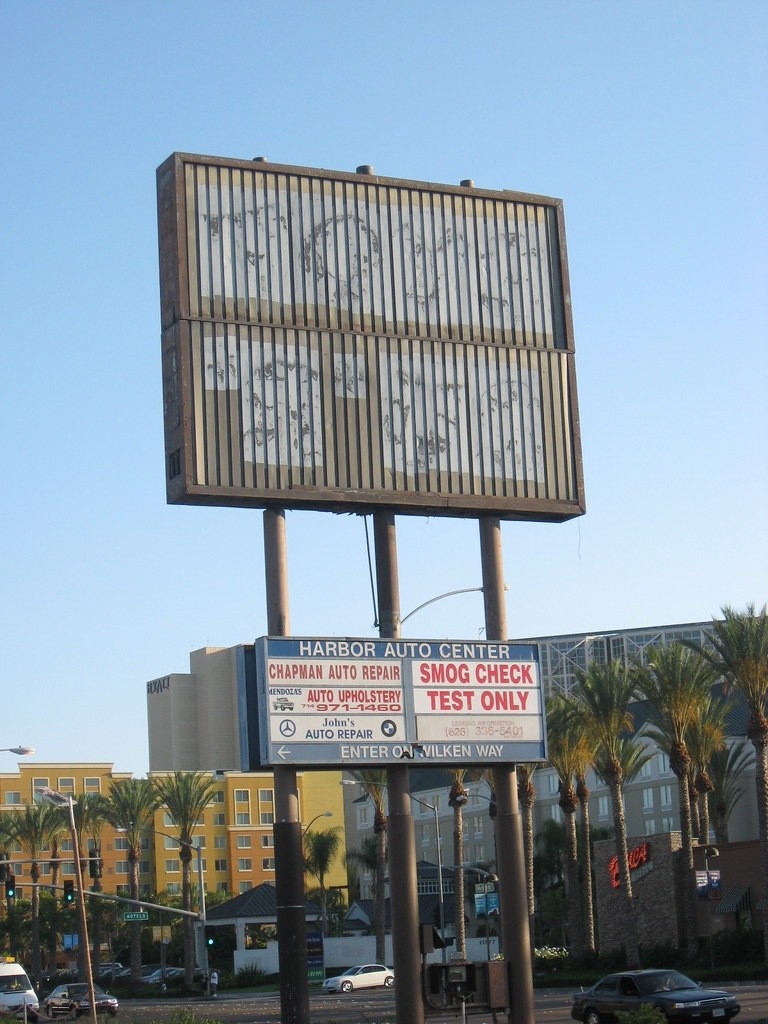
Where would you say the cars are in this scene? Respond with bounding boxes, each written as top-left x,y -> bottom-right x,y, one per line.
97,961 -> 218,993
44,983 -> 118,1017
322,964 -> 393,994
571,969 -> 740,1023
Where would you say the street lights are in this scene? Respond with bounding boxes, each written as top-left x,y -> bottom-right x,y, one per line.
115,828 -> 213,1000
704,847 -> 721,975
340,780 -> 445,1008
38,785 -> 98,1024
484,873 -> 499,960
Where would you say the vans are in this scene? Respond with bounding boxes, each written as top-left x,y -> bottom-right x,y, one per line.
0,957 -> 40,1024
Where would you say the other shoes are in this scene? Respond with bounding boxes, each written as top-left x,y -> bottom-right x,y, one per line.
213,994 -> 216,997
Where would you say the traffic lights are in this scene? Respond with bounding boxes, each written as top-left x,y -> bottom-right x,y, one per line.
6,874 -> 16,899
206,924 -> 216,949
63,880 -> 75,904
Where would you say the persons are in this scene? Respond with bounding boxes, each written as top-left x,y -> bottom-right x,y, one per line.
9,978 -> 22,990
208,968 -> 219,998
